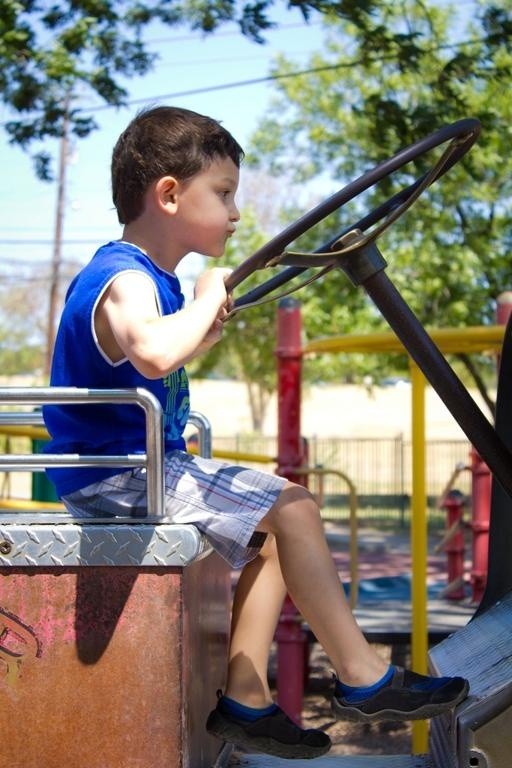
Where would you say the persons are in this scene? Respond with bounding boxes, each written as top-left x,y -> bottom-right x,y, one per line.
40,104 -> 475,759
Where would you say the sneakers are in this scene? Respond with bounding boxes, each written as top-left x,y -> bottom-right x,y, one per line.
203,687 -> 332,760
328,664 -> 470,724
